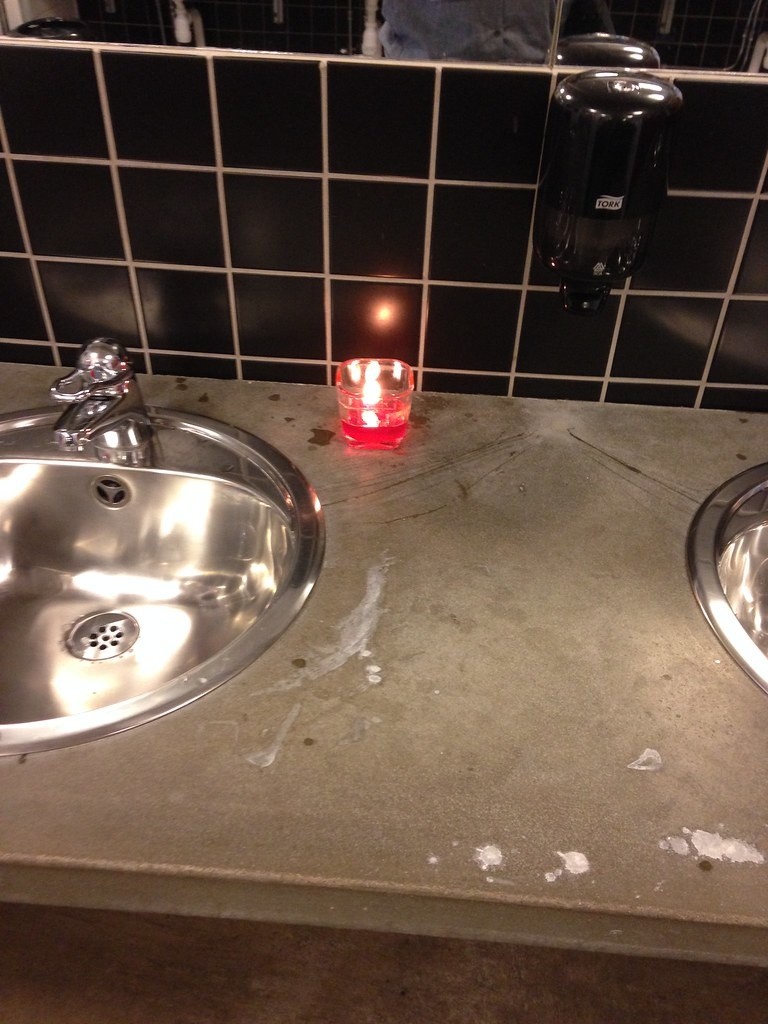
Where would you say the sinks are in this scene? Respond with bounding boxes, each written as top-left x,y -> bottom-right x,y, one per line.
685,460 -> 768,695
0,401 -> 326,756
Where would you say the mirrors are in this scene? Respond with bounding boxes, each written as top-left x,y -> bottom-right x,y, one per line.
0,0 -> 768,78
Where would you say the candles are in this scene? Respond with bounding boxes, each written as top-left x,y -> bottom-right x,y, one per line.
337,358 -> 414,449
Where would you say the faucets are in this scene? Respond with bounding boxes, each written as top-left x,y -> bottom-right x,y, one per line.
47,337 -> 155,467
